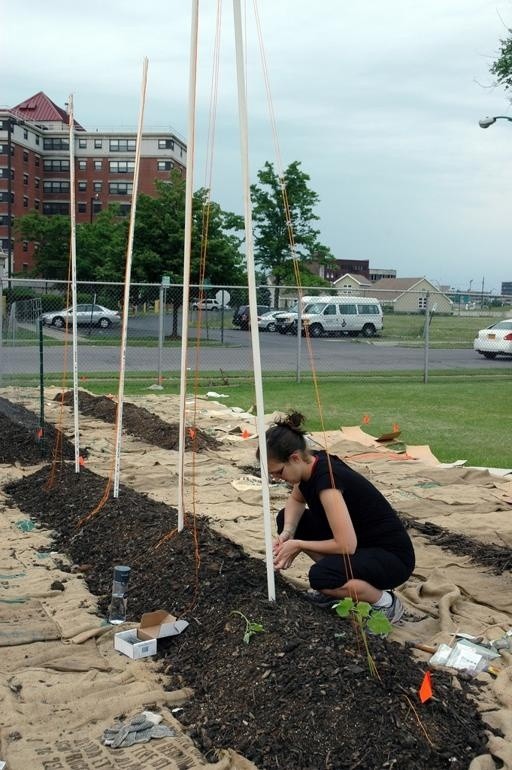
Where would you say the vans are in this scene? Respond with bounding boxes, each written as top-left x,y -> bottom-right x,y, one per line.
275,296 -> 383,337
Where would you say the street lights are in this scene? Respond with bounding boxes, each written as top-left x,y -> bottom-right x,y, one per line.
2,119 -> 14,316
91,193 -> 101,224
479,116 -> 512,128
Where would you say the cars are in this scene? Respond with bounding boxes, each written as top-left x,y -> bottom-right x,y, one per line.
474,319 -> 512,358
38,304 -> 120,328
192,299 -> 231,311
232,305 -> 286,331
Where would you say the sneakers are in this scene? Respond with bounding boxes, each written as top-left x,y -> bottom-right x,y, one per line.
362,591 -> 405,640
307,590 -> 344,608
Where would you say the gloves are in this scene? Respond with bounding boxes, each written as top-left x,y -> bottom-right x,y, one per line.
102,711 -> 176,749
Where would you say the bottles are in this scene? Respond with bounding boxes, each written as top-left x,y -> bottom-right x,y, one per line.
106,564 -> 132,625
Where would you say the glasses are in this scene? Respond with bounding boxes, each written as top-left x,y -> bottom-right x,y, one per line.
268,464 -> 287,476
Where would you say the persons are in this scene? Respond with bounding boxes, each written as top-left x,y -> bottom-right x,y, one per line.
256,409 -> 417,637
